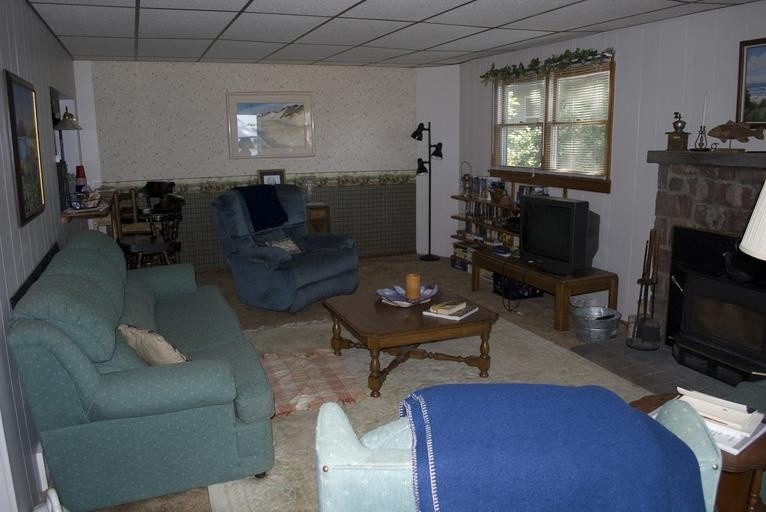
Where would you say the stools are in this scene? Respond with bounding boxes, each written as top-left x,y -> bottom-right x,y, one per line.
627,392 -> 765,512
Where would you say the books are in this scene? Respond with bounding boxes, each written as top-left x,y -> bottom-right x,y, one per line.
646,385 -> 766,455
422,298 -> 480,322
472,174 -> 501,200
464,221 -> 519,252
464,202 -> 505,226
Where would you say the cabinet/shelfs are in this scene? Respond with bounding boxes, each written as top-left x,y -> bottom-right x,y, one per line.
449,194 -> 520,270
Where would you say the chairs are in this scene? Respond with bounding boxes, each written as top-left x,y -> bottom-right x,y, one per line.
112,188 -> 182,270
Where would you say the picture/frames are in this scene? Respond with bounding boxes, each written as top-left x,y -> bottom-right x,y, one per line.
256,168 -> 287,187
735,37 -> 766,129
225,90 -> 315,159
0,68 -> 47,229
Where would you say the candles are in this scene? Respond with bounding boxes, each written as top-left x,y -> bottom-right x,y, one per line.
702,95 -> 707,127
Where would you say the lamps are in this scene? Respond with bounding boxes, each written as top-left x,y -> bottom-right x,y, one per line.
53,105 -> 83,163
410,121 -> 444,262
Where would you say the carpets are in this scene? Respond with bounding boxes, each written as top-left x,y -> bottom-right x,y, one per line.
207,316 -> 655,512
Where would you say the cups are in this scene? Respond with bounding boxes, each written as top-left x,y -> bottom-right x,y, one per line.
405,274 -> 420,300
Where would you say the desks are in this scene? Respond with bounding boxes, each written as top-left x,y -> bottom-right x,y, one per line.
471,247 -> 619,332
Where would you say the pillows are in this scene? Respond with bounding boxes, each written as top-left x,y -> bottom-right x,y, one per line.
264,237 -> 302,255
118,324 -> 190,367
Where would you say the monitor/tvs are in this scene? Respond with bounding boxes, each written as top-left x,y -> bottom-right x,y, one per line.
518,194 -> 600,277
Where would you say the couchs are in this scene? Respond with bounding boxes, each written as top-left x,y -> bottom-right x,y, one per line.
315,381 -> 722,511
210,182 -> 360,315
5,228 -> 276,512
